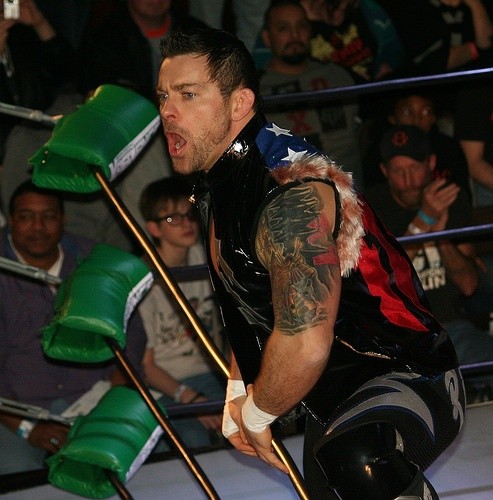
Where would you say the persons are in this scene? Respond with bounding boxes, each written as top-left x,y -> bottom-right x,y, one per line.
157,20 -> 466,500
0,0 -> 493,482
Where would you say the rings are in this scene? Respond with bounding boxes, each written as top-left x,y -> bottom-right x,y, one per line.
50,437 -> 60,445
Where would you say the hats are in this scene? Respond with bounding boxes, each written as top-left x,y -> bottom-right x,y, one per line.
381,123 -> 431,165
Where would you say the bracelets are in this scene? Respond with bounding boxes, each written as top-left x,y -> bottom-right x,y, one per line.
417,210 -> 438,225
16,419 -> 35,441
408,224 -> 426,235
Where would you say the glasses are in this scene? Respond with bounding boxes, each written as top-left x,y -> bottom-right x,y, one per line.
401,106 -> 434,118
153,209 -> 200,226
